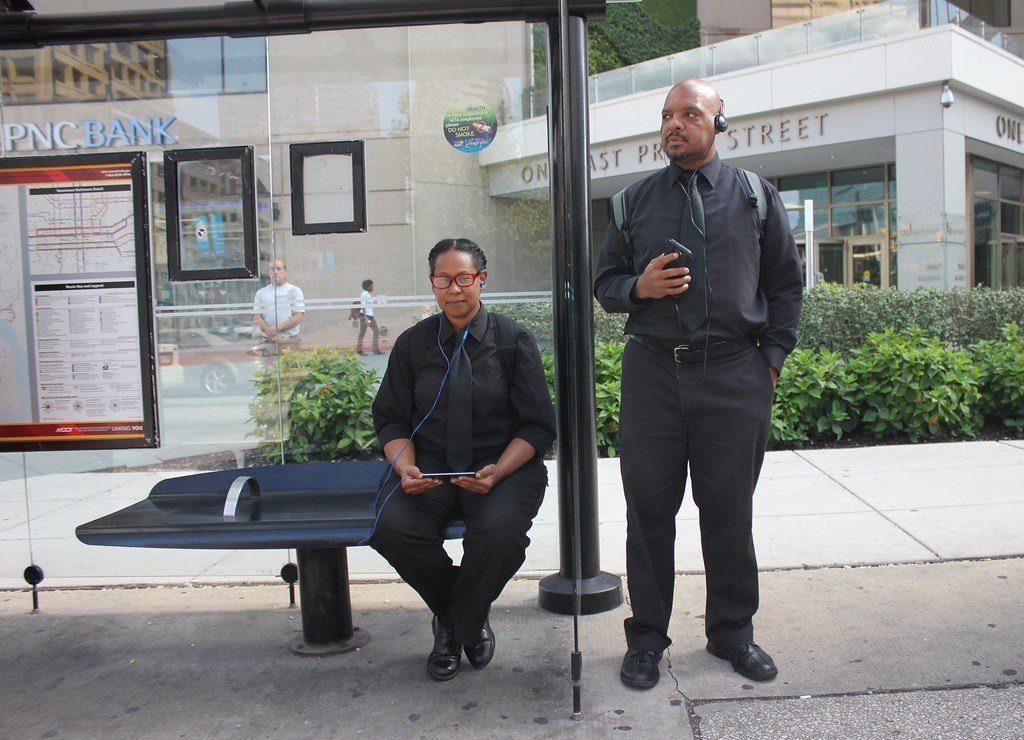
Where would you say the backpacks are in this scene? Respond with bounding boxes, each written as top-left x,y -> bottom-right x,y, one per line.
351,301 -> 360,319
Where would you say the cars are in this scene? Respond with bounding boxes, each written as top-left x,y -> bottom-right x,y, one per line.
160,328 -> 276,397
160,345 -> 183,387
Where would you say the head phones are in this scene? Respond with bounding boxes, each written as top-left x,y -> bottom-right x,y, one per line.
715,99 -> 728,132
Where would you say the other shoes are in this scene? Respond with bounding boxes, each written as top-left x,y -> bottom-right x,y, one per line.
358,351 -> 369,356
374,350 -> 385,354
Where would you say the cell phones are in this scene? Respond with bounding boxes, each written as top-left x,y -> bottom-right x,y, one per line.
423,471 -> 476,480
662,238 -> 692,288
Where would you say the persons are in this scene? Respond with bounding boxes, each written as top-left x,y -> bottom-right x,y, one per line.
374,239 -> 557,682
592,78 -> 803,687
356,279 -> 385,355
254,260 -> 305,437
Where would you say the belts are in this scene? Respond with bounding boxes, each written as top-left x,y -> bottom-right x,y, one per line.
631,333 -> 753,365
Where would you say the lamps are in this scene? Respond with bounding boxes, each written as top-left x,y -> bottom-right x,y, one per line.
940,84 -> 955,109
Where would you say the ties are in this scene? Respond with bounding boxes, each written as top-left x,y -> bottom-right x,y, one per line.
441,333 -> 475,473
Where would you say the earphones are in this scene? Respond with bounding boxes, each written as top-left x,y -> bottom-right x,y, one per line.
480,281 -> 484,287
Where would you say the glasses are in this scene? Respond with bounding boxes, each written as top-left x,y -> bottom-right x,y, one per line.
431,271 -> 481,288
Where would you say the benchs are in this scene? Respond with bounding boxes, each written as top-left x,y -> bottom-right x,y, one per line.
77,458 -> 466,658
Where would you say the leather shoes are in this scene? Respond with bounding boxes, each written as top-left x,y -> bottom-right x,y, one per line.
707,641 -> 779,681
426,613 -> 464,681
464,616 -> 496,667
620,648 -> 663,689
